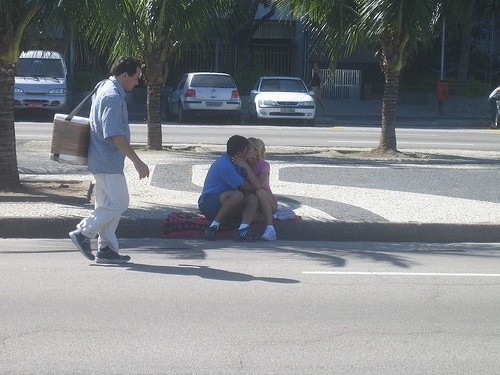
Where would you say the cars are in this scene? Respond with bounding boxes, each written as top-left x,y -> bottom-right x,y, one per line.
165,71 -> 243,123
247,75 -> 317,125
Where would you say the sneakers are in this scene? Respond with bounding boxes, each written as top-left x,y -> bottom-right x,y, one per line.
95,247 -> 131,263
69,229 -> 95,261
272,208 -> 296,221
234,226 -> 258,242
261,225 -> 276,241
205,225 -> 220,240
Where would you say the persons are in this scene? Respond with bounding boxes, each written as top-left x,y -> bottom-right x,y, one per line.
198,135 -> 278,242
68,55 -> 150,264
488,86 -> 500,129
308,62 -> 326,115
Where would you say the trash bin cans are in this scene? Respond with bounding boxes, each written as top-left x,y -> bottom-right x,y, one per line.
436,79 -> 448,103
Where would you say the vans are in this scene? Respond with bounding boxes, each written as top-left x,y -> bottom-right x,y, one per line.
10,49 -> 72,118
